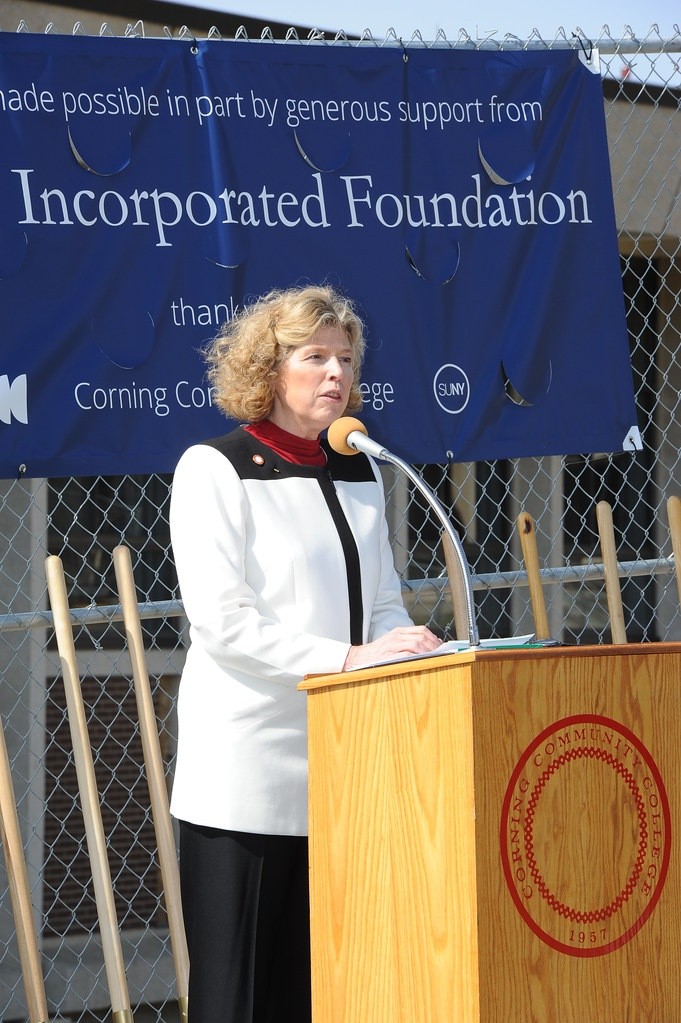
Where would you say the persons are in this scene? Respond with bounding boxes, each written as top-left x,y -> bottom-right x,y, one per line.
166,286 -> 445,1022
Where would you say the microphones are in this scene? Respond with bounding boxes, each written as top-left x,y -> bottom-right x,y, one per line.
328,417 -> 480,646
